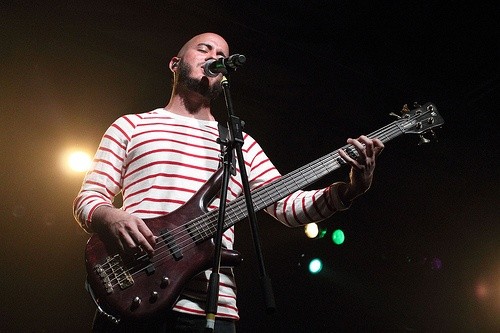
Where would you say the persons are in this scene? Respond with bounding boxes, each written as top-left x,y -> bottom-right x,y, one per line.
73,33 -> 384,333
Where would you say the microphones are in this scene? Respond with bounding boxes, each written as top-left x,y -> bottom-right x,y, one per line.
204,54 -> 246,77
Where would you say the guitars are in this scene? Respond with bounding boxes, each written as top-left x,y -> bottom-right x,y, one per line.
84,91 -> 446,326
